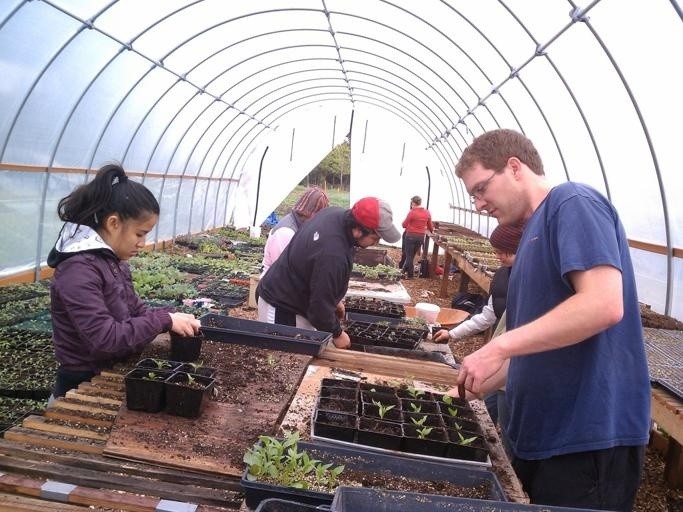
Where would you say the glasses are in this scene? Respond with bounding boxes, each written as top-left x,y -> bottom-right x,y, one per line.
469,165 -> 505,203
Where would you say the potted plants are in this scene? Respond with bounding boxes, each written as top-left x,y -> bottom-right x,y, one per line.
242,436 -> 508,512
125,357 -> 218,416
312,377 -> 492,459
169,330 -> 204,361
337,295 -> 449,349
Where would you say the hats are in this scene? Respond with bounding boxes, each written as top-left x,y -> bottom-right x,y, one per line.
489,223 -> 524,254
291,188 -> 330,219
351,198 -> 401,243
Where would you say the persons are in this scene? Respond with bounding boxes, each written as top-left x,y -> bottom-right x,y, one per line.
396,194 -> 436,280
254,197 -> 402,349
432,221 -> 525,429
258,185 -> 349,323
44,161 -> 203,398
446,128 -> 652,507
398,202 -> 412,269
